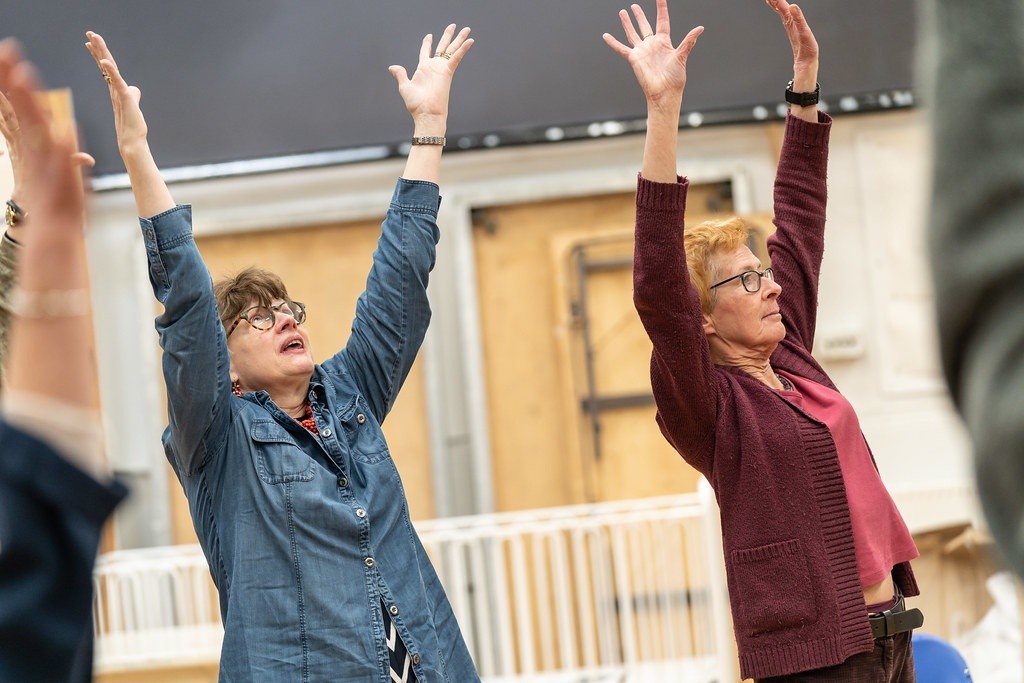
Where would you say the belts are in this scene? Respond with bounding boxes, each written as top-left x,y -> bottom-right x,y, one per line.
864,595 -> 923,642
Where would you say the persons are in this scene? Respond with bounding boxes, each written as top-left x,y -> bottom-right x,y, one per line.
0,36 -> 126,682
601,0 -> 924,682
84,23 -> 475,683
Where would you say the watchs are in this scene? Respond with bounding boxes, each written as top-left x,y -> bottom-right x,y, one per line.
785,76 -> 821,106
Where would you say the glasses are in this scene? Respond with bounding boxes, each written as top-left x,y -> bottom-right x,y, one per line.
225,301 -> 307,335
711,268 -> 776,293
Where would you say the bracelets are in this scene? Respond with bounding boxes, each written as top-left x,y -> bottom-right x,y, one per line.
5,284 -> 91,320
411,136 -> 446,146
4,200 -> 30,228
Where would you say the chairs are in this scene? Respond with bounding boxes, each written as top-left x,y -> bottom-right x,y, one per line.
911,634 -> 973,683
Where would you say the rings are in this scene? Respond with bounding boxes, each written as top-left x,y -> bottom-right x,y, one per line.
9,126 -> 20,135
102,72 -> 111,82
433,52 -> 444,57
444,52 -> 451,59
642,33 -> 652,39
